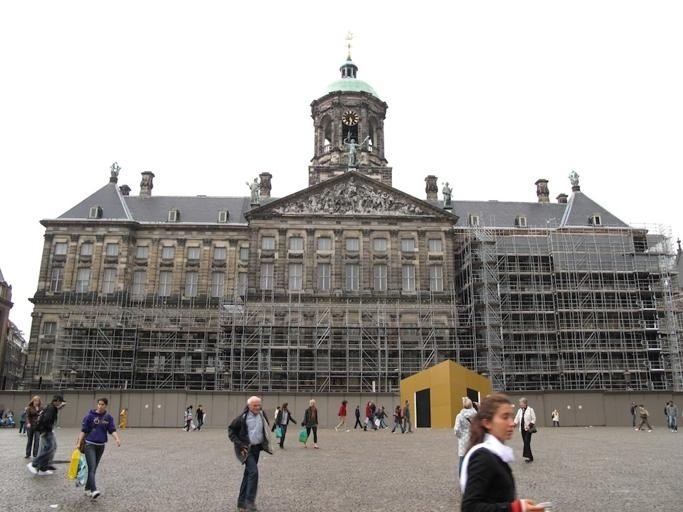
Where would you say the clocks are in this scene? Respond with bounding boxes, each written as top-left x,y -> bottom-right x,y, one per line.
342,110 -> 359,127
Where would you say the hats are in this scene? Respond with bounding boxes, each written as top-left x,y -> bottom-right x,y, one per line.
52,394 -> 66,402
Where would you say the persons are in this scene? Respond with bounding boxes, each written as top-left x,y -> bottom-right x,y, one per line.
343,138 -> 365,168
246,178 -> 262,203
228,396 -> 320,512
185,405 -> 205,432
552,409 -> 559,427
453,393 -> 537,512
443,183 -> 453,207
336,400 -> 413,435
0,394 -> 127,500
631,400 -> 678,430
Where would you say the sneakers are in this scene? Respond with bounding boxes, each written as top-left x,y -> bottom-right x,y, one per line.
26,463 -> 36,474
84,489 -> 100,500
38,469 -> 53,476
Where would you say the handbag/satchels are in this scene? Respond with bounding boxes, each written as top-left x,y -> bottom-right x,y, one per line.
75,452 -> 86,487
374,419 -> 381,427
275,425 -> 282,439
76,436 -> 85,452
67,449 -> 80,480
528,426 -> 537,433
298,429 -> 308,442
